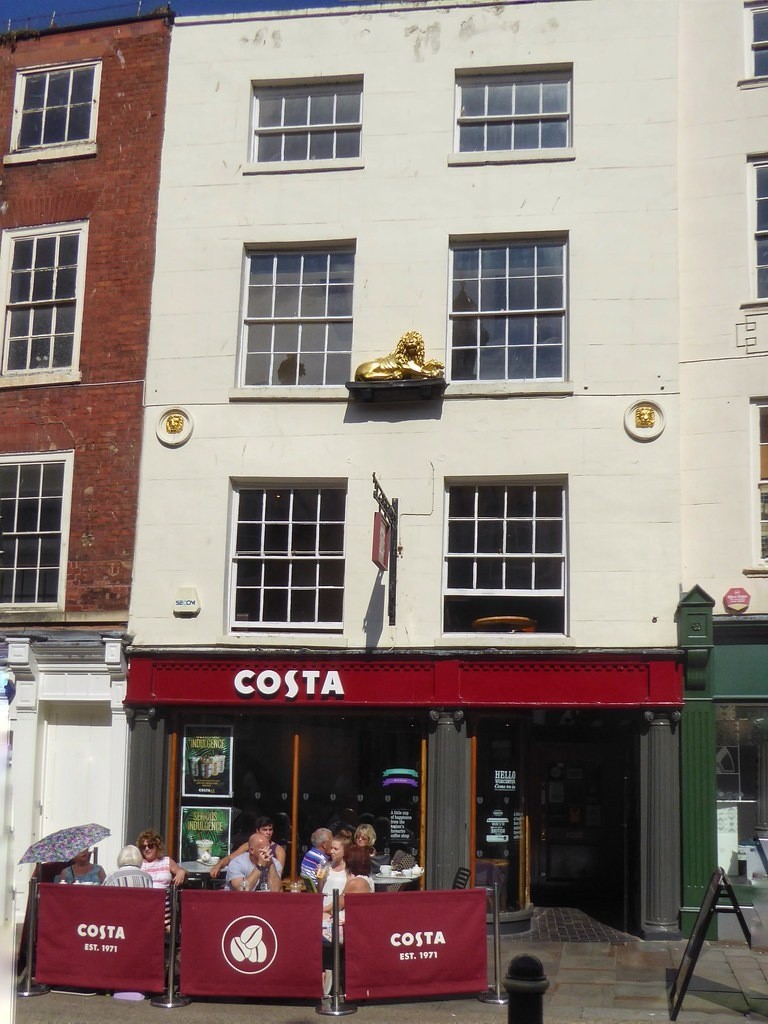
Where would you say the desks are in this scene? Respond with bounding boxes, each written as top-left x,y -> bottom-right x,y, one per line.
177,862 -> 227,889
387,839 -> 416,865
373,865 -> 424,892
472,615 -> 538,632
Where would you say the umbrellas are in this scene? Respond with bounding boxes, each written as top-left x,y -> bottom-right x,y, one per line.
18,825 -> 110,883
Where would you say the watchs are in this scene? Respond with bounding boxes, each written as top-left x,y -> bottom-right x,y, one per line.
256,864 -> 265,871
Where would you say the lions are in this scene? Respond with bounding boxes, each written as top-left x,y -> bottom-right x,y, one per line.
636,407 -> 655,425
355,331 -> 444,381
166,416 -> 183,433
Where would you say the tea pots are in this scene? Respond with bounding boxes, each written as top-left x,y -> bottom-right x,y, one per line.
409,864 -> 424,875
201,851 -> 211,863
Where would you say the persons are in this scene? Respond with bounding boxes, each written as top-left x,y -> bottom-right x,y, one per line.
328,808 -> 414,855
272,812 -> 301,852
136,809 -> 400,994
100,845 -> 154,888
60,848 -> 105,884
238,809 -> 259,846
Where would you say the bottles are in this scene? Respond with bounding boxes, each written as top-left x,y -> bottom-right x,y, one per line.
58,880 -> 68,884
72,878 -> 81,884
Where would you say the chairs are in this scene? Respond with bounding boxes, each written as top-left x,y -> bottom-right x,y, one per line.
452,867 -> 471,889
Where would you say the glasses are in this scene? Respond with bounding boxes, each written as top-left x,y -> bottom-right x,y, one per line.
357,835 -> 366,840
139,844 -> 155,851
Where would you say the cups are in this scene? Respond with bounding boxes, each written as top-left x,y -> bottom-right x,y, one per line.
260,883 -> 271,892
380,865 -> 392,875
240,880 -> 250,892
402,870 -> 412,877
316,858 -> 330,879
82,882 -> 93,885
196,840 -> 213,859
290,881 -> 302,892
209,857 -> 219,864
188,755 -> 226,778
